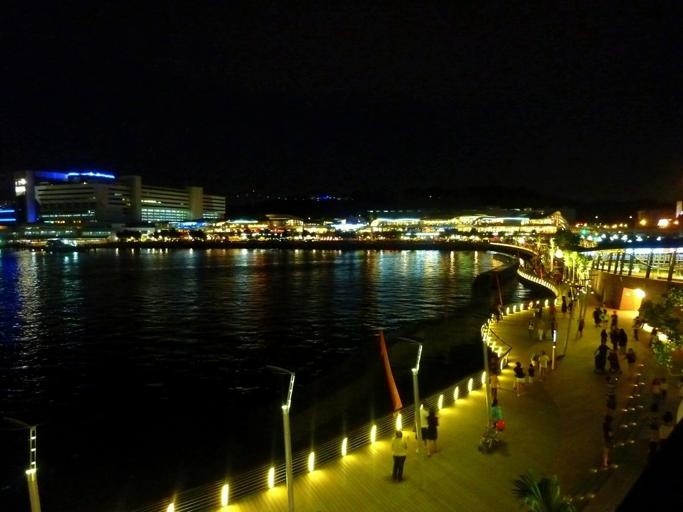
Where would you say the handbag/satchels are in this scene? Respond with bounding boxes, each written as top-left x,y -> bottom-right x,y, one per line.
496,420 -> 505,431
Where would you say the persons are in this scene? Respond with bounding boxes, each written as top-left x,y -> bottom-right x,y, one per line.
488,369 -> 499,406
592,305 -> 675,471
390,429 -> 407,482
526,284 -> 585,343
513,349 -> 550,398
413,401 -> 428,455
424,407 -> 439,453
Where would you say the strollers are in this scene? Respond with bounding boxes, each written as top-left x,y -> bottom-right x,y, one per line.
478,430 -> 501,455
